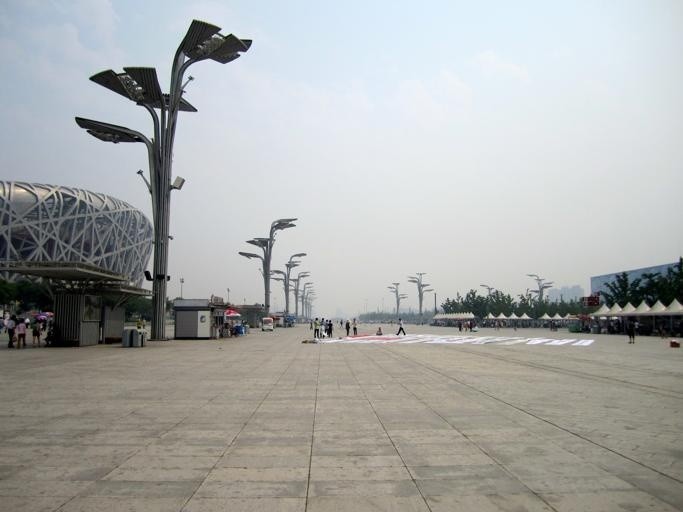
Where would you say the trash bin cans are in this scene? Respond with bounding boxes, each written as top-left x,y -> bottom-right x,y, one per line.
121,328 -> 148,347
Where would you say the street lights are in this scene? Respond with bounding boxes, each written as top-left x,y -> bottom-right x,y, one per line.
237,216 -> 317,322
71,18 -> 251,340
385,270 -> 557,326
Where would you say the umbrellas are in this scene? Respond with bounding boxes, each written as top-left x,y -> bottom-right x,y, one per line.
224,309 -> 240,317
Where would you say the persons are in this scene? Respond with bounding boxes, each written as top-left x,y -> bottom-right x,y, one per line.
0,310 -> 53,349
344,319 -> 350,336
375,327 -> 382,335
626,321 -> 634,344
395,318 -> 405,335
351,318 -> 358,335
309,317 -> 334,338
339,320 -> 343,329
136,316 -> 145,329
455,319 -> 682,334
